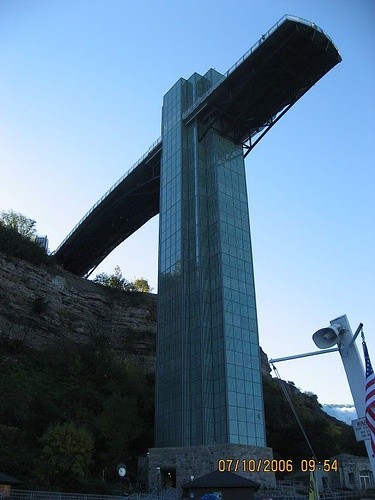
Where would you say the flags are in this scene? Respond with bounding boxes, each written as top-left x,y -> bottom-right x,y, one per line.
361,342 -> 374,458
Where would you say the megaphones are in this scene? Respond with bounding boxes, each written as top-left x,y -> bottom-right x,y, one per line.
312,323 -> 342,350
